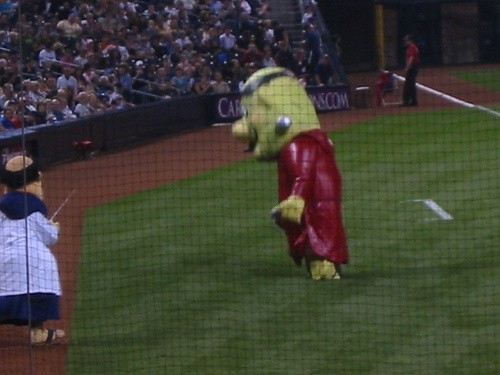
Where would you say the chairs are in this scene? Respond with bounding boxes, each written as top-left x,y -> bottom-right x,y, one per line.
373,69 -> 406,108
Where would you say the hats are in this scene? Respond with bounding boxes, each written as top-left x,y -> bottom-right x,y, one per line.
401,34 -> 413,41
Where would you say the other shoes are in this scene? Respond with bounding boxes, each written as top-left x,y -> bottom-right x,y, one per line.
400,102 -> 417,107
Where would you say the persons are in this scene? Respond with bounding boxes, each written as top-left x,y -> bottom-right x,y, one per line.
400,32 -> 422,107
0,155 -> 67,347
0,0 -> 388,156
